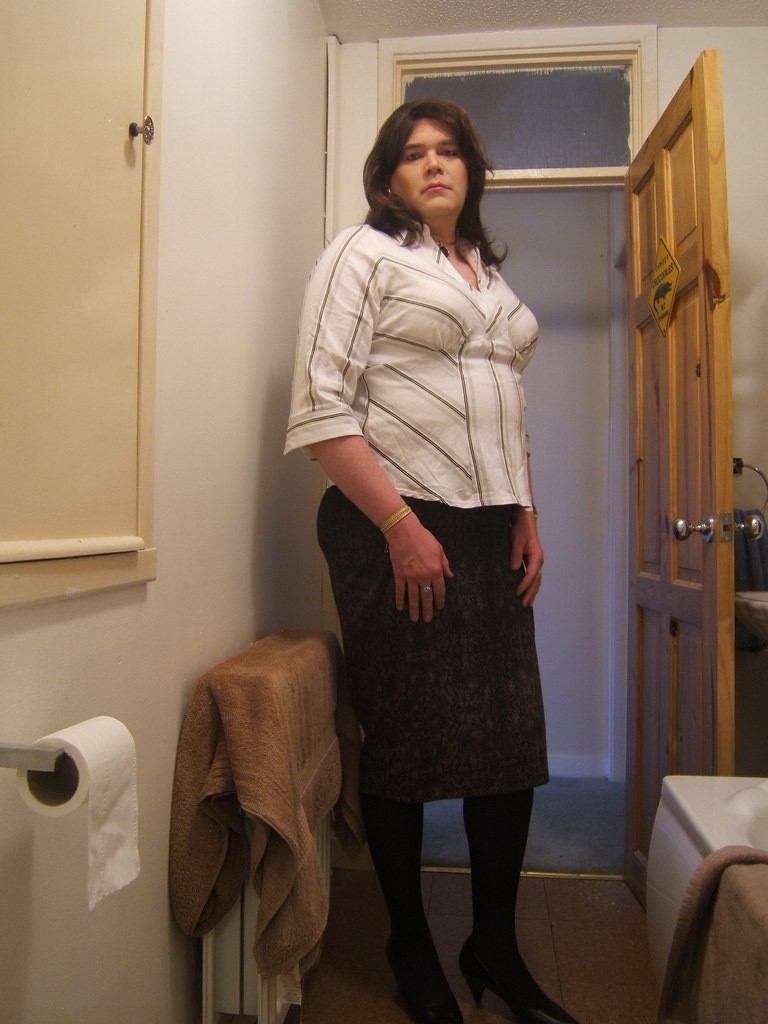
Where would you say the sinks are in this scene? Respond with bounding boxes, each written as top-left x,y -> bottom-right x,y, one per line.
735,592 -> 768,641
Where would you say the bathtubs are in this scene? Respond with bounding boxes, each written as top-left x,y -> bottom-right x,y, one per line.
643,770 -> 767,982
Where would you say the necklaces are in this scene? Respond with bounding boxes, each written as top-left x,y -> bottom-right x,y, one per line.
436,241 -> 455,259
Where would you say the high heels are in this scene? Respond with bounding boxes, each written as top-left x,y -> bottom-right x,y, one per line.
386,938 -> 464,1024
458,936 -> 579,1024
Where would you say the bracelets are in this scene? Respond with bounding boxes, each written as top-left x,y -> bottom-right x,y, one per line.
380,505 -> 413,533
533,504 -> 538,521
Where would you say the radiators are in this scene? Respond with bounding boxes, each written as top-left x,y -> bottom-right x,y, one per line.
172,621 -> 371,1024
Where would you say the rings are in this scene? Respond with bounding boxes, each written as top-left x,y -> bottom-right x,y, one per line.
421,587 -> 432,592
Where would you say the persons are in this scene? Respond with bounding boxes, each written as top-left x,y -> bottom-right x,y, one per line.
283,99 -> 579,1024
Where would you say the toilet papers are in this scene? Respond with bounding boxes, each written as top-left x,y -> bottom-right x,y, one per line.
20,715 -> 141,912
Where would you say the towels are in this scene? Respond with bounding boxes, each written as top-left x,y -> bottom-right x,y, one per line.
736,509 -> 768,592
650,844 -> 767,1024
166,622 -> 360,977
